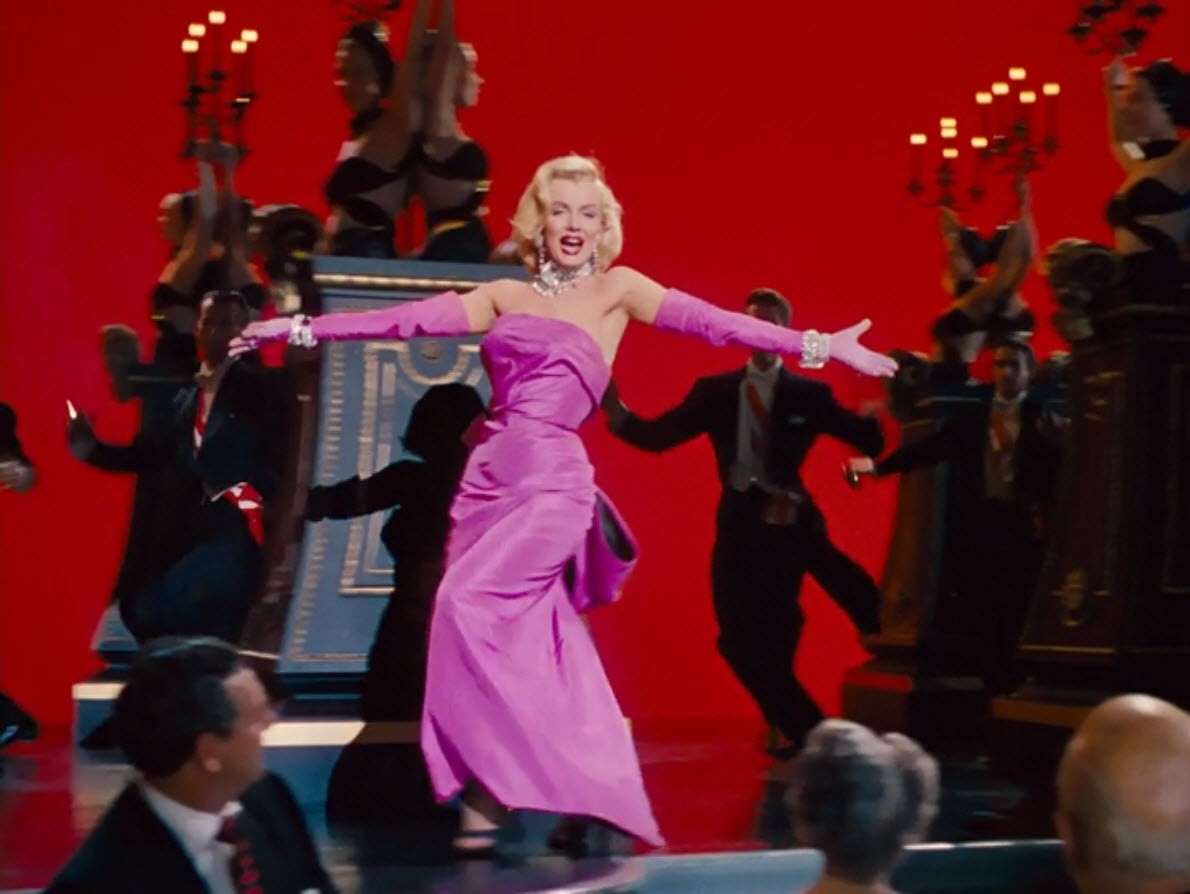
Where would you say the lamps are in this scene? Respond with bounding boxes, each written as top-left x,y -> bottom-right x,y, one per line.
907,64 -> 1064,210
179,6 -> 259,164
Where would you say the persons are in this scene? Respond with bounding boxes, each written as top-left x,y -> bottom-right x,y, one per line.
840,58 -> 1190,721
229,153 -> 898,856
594,279 -> 885,758
787,716 -> 937,894
1053,692 -> 1189,894
57,178 -> 320,771
0,402 -> 40,748
39,636 -> 342,894
318,1 -> 502,263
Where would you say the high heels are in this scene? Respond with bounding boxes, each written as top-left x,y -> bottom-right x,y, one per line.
545,815 -> 591,851
430,807 -> 523,866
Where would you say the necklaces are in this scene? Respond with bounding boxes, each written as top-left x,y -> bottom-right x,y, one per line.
533,259 -> 596,299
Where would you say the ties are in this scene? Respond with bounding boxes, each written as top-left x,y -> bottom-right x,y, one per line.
216,817 -> 266,894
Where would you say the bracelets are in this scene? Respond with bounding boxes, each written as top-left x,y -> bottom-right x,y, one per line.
797,328 -> 831,369
285,313 -> 318,349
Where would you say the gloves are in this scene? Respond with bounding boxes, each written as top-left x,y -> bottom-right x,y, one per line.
228,289 -> 475,362
652,286 -> 900,378
